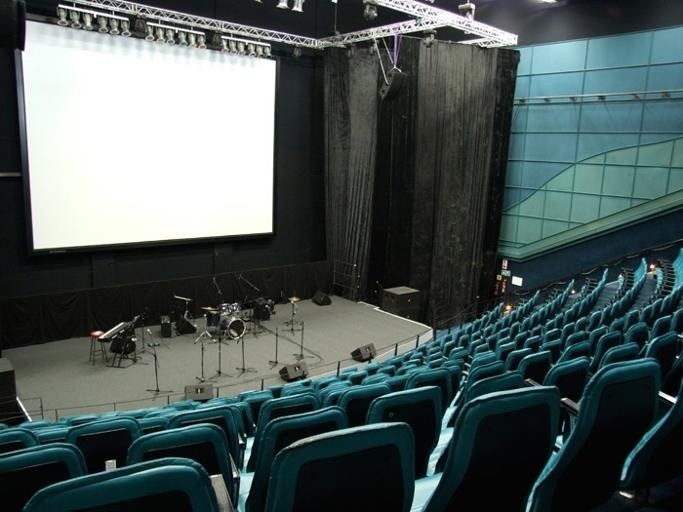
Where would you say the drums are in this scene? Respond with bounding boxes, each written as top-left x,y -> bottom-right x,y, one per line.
206,311 -> 219,329
222,318 -> 246,340
220,304 -> 232,316
231,303 -> 240,313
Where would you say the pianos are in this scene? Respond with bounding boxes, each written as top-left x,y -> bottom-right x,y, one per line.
98,321 -> 130,342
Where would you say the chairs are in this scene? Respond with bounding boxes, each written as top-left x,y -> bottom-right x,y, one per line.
411,386 -> 561,512
367,251 -> 682,477
526,357 -> 661,512
616,385 -> 683,512
264,421 -> 416,511
0,441 -> 90,512
23,457 -> 237,511
0,355 -> 391,479
244,405 -> 351,511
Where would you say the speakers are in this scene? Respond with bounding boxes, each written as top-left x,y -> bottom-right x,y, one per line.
175,315 -> 197,335
379,70 -> 407,102
279,360 -> 309,382
382,286 -> 421,322
312,288 -> 332,305
0,357 -> 16,426
185,383 -> 214,401
350,343 -> 378,362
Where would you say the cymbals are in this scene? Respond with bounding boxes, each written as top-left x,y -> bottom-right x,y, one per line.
288,297 -> 301,302
201,306 -> 219,310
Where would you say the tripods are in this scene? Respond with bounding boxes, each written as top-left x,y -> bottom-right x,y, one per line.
293,321 -> 315,361
145,348 -> 174,401
281,305 -> 302,336
268,328 -> 285,370
236,335 -> 258,378
131,323 -> 153,356
196,344 -> 218,385
194,314 -> 216,343
208,337 -> 233,380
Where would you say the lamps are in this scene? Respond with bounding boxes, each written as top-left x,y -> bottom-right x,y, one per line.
55,1 -> 305,60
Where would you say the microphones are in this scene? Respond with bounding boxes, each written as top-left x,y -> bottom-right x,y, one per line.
147,328 -> 152,335
